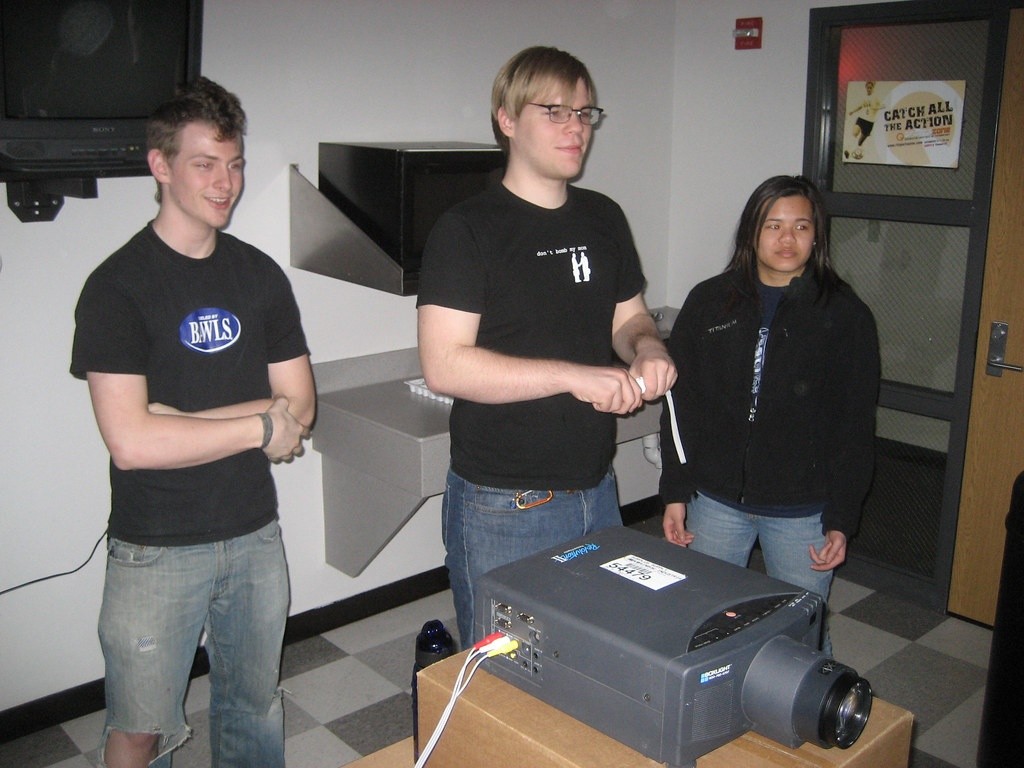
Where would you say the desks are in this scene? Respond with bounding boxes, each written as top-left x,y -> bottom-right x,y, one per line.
313,330 -> 672,579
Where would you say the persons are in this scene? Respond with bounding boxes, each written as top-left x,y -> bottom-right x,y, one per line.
418,45 -> 679,653
70,73 -> 319,767
658,175 -> 883,658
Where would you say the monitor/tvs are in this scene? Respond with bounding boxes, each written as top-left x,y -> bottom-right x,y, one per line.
0,0 -> 205,173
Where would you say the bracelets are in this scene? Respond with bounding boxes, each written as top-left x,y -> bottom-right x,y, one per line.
256,412 -> 274,449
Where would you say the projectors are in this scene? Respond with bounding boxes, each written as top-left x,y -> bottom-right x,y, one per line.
473,526 -> 872,768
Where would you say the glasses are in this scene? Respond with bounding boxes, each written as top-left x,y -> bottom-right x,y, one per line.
526,102 -> 604,127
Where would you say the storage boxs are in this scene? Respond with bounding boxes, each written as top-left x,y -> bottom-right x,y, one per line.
414,647 -> 916,768
